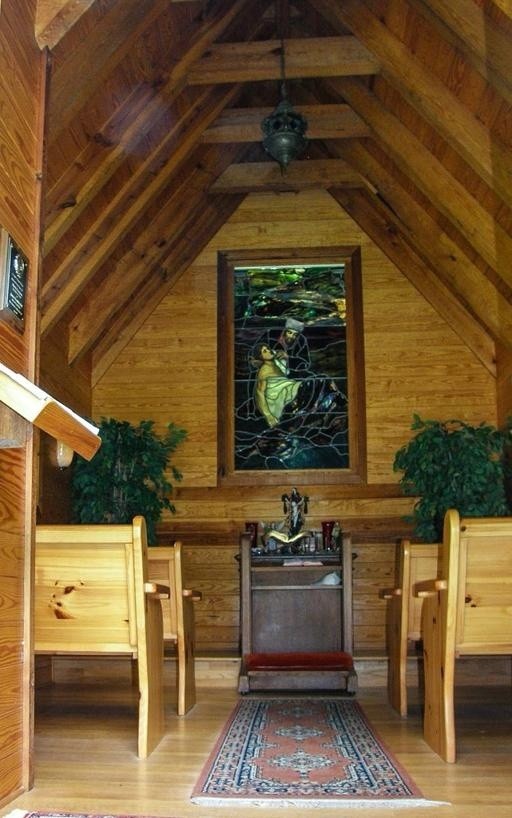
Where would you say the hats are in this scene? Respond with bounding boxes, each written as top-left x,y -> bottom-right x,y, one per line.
284,316 -> 305,333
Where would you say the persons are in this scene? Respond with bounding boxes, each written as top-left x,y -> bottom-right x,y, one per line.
248,318 -> 313,378
253,342 -> 347,429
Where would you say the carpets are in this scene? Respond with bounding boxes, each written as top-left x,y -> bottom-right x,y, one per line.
1,808 -> 184,818
189,698 -> 451,808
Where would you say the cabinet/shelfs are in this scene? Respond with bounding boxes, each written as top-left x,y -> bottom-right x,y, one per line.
238,532 -> 357,695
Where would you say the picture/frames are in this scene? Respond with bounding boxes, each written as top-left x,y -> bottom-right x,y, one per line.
216,245 -> 367,488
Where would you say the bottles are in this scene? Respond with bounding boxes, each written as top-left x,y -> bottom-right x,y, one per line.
267,523 -> 276,553
332,522 -> 341,549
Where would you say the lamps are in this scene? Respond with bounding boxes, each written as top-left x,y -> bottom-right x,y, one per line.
260,39 -> 308,177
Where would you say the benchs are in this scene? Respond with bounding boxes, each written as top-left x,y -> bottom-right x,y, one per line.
378,540 -> 438,718
412,508 -> 512,763
147,539 -> 202,715
34,515 -> 170,758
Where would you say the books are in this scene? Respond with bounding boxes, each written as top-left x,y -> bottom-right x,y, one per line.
283,559 -> 324,566
